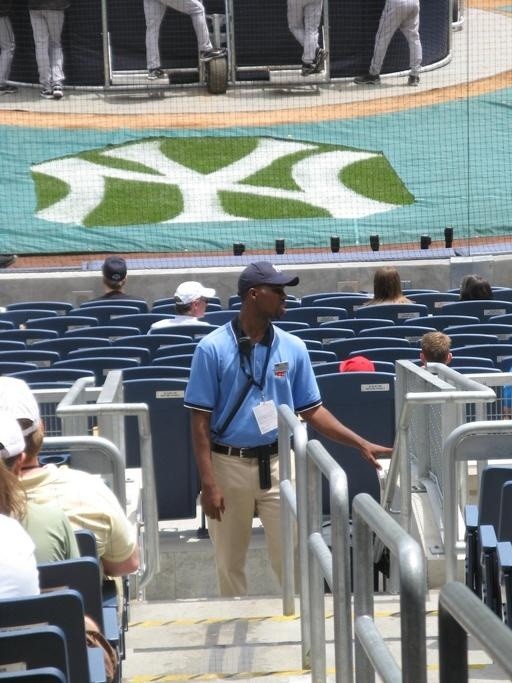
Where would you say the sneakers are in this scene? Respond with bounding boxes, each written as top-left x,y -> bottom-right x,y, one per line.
0,85 -> 18,93
201,48 -> 226,62
147,69 -> 163,79
40,84 -> 63,98
301,48 -> 328,75
408,76 -> 419,86
354,73 -> 380,85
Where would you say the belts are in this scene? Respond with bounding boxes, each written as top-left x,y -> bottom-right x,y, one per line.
210,437 -> 293,457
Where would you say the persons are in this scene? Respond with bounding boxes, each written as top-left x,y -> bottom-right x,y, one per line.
26,1 -> 72,106
146,281 -> 216,336
364,268 -> 414,309
142,1 -> 229,80
353,0 -> 424,85
459,274 -> 493,301
0,375 -> 141,576
184,261 -> 397,597
0,255 -> 17,269
500,386 -> 512,420
86,256 -> 145,302
0,412 -> 80,566
0,0 -> 21,95
285,0 -> 330,77
0,510 -> 121,683
420,332 -> 453,367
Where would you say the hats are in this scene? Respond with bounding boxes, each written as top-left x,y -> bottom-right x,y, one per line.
238,261 -> 299,292
175,281 -> 215,305
0,376 -> 39,459
105,257 -> 126,281
